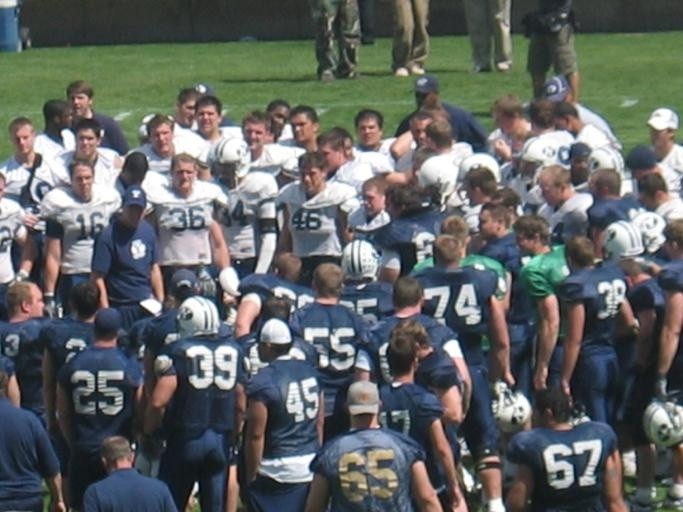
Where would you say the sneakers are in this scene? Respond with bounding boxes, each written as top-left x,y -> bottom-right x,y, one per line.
320,68 -> 335,80
411,62 -> 426,75
395,67 -> 409,76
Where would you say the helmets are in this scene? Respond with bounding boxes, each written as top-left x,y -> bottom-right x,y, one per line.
341,237 -> 380,285
640,396 -> 683,449
589,143 -> 624,173
633,210 -> 667,254
601,220 -> 645,257
210,135 -> 252,178
176,295 -> 221,338
492,385 -> 533,434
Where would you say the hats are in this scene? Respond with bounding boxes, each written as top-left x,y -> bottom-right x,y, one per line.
120,184 -> 148,209
169,268 -> 198,290
646,106 -> 679,132
345,379 -> 380,415
252,317 -> 293,347
512,134 -> 557,163
542,73 -> 569,104
94,306 -> 123,333
408,74 -> 439,95
626,145 -> 658,169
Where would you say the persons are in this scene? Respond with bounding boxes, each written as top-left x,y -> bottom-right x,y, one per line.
0,73 -> 683,512
305,0 -> 363,80
521,0 -> 580,109
388,0 -> 430,75
463,1 -> 513,72
